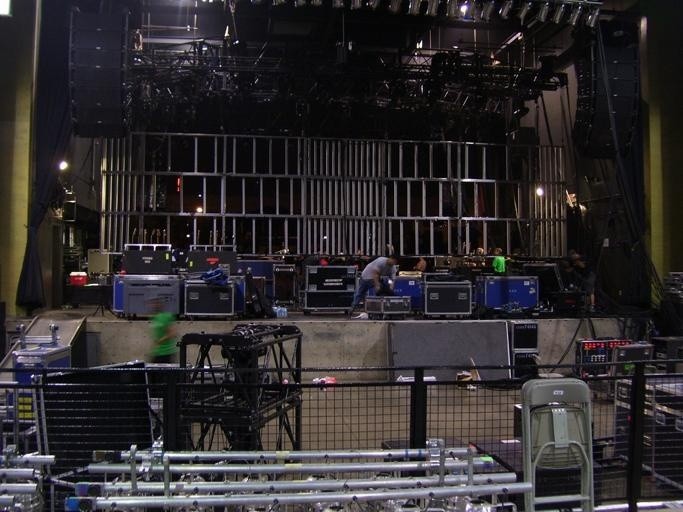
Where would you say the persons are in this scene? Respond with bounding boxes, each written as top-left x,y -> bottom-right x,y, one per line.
491,246 -> 506,275
566,248 -> 597,313
200,255 -> 226,282
141,292 -> 180,364
346,253 -> 399,321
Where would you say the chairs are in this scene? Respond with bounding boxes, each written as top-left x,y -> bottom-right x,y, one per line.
521,378 -> 596,512
